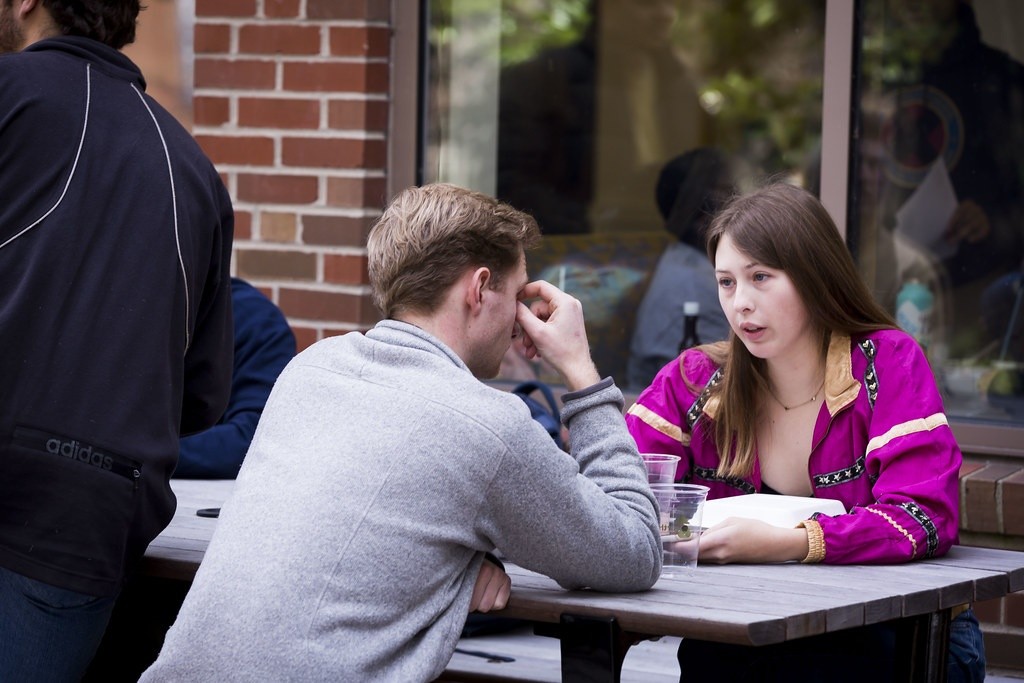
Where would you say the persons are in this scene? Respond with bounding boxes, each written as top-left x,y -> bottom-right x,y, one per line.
172,273 -> 299,481
625,147 -> 727,391
615,183 -> 992,683
134,180 -> 666,683
0,1 -> 235,683
867,1 -> 1024,383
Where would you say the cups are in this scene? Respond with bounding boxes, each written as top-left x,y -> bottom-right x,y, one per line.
641,453 -> 682,539
647,482 -> 711,582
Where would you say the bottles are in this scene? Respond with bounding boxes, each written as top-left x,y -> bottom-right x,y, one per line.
678,299 -> 702,357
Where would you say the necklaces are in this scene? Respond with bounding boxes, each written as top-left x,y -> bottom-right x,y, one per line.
763,377 -> 827,412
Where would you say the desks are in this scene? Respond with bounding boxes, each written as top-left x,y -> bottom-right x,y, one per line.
142,478 -> 1024,683
